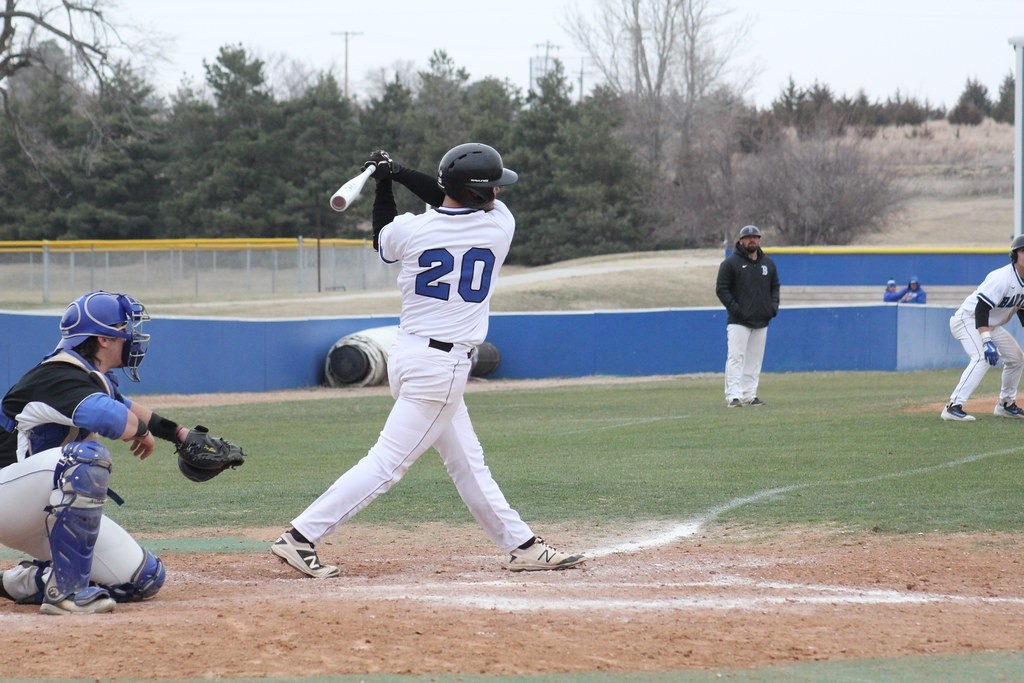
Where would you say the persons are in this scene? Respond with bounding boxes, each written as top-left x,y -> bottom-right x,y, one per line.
1,290 -> 246,618
885,275 -> 926,305
940,235 -> 1024,421
268,142 -> 588,579
715,224 -> 780,408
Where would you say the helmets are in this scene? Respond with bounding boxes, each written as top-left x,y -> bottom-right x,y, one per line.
436,143 -> 521,190
55,291 -> 143,351
739,226 -> 761,238
1011,235 -> 1024,251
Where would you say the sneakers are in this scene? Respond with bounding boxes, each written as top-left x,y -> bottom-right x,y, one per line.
994,400 -> 1024,418
270,530 -> 342,579
940,402 -> 976,422
508,536 -> 589,572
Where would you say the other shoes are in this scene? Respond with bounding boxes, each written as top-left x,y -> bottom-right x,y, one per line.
728,398 -> 742,408
748,397 -> 768,407
40,593 -> 118,615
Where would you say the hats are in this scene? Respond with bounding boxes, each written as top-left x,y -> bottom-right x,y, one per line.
887,278 -> 896,286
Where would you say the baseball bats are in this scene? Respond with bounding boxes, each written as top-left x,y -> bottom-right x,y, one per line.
330,165 -> 376,213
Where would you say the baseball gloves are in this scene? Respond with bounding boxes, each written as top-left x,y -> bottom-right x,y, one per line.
177,424 -> 246,482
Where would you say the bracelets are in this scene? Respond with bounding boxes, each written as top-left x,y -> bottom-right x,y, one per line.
136,430 -> 150,438
176,426 -> 184,437
